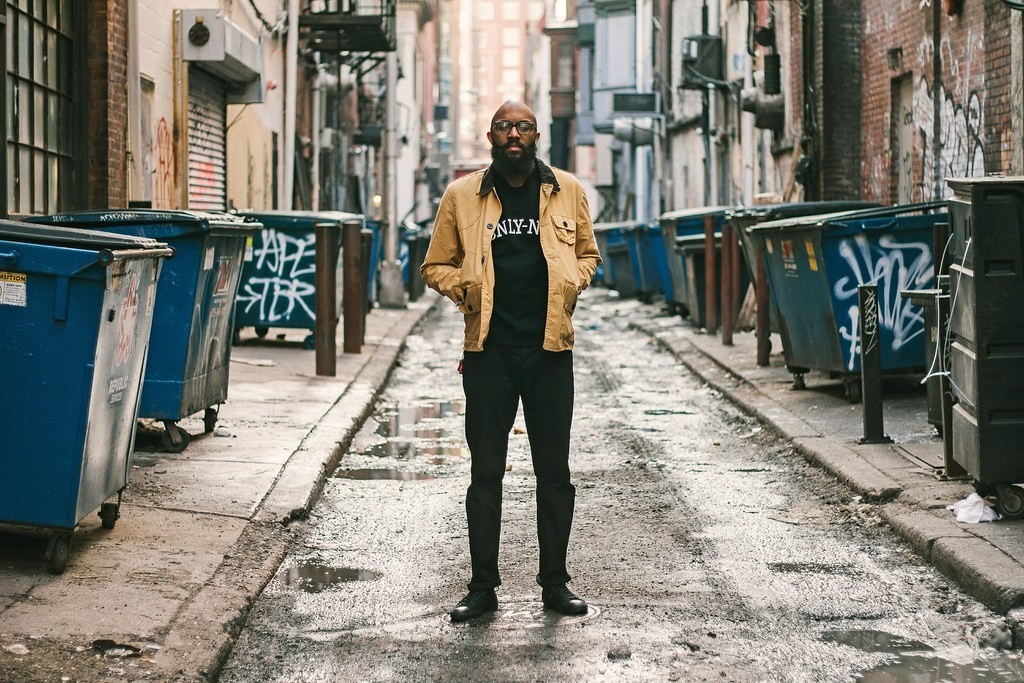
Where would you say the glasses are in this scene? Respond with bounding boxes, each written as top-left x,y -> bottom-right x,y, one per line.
491,121 -> 537,135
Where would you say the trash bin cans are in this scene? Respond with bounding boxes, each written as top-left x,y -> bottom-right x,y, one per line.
590,220 -> 675,319
229,210 -> 364,348
18,209 -> 264,452
945,174 -> 1024,519
732,200 -> 883,332
366,220 -> 432,310
674,228 -> 724,329
658,206 -> 745,326
0,217 -> 176,571
746,200 -> 950,402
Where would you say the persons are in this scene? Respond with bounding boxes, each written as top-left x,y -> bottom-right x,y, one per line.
420,99 -> 605,621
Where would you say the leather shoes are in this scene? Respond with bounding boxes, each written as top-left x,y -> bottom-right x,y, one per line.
541,585 -> 588,615
450,589 -> 499,620
